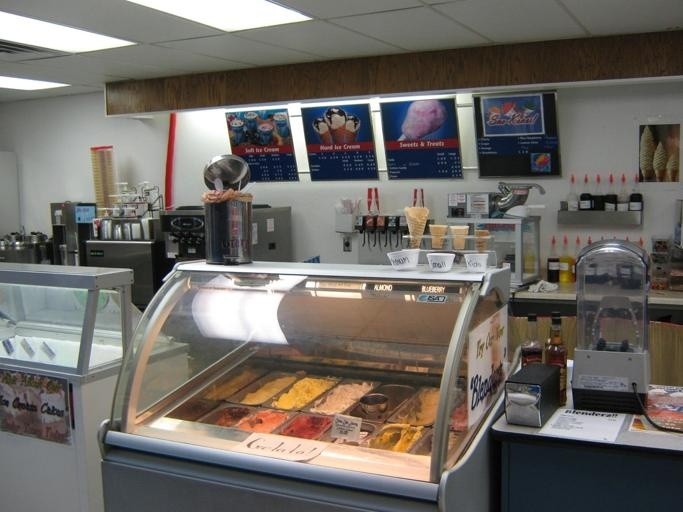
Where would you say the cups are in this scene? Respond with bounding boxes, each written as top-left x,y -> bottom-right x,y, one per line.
360,392 -> 391,419
462,253 -> 488,272
227,112 -> 288,146
387,249 -> 418,270
427,252 -> 454,273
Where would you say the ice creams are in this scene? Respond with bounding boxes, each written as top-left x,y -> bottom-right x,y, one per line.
313,107 -> 360,143
0,372 -> 66,441
640,126 -> 678,182
488,102 -> 536,121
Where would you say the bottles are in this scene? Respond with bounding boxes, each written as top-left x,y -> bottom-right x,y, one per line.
546,231 -> 644,287
521,312 -> 566,404
0,231 -> 43,251
565,173 -> 641,212
96,217 -> 152,240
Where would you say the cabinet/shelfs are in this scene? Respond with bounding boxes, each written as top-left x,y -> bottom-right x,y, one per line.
442,216 -> 543,285
0,262 -> 189,512
99,260 -> 514,512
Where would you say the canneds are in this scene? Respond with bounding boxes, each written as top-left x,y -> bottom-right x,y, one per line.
274,113 -> 290,137
258,124 -> 274,145
244,112 -> 257,132
231,121 -> 245,143
